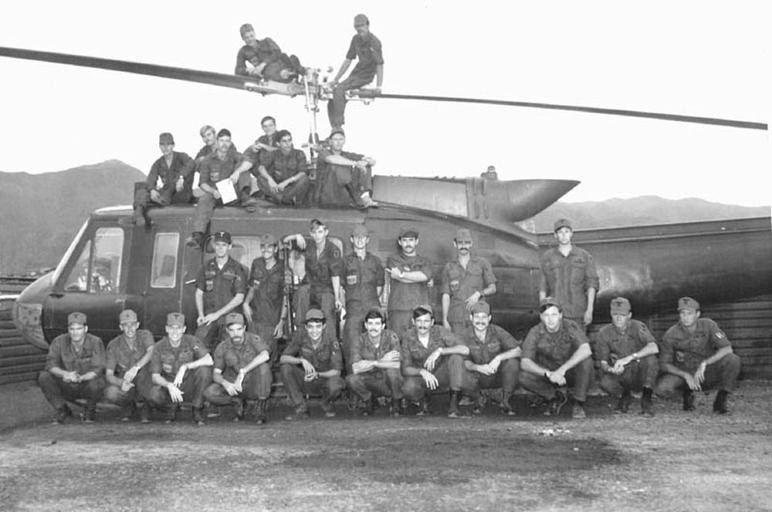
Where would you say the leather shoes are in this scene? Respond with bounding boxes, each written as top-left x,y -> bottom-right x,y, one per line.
352,197 -> 381,212
244,192 -> 298,212
186,231 -> 204,250
119,191 -> 169,228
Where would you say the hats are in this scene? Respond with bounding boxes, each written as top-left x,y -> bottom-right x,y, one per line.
556,217 -> 574,230
355,15 -> 369,25
210,219 -> 472,245
68,297 -> 699,327
330,128 -> 343,138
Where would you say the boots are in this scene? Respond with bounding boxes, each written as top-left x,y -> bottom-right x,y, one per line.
619,392 -> 632,412
640,387 -> 652,415
55,385 -> 588,422
682,389 -> 694,410
714,390 -> 729,411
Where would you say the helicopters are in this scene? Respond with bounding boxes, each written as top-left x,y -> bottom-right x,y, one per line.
0,47 -> 772,352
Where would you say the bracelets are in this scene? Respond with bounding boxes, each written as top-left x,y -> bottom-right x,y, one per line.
184,364 -> 191,371
280,316 -> 288,321
376,86 -> 382,90
478,289 -> 486,297
632,353 -> 639,362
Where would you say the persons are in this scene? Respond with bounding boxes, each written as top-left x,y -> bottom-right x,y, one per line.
400,303 -> 474,419
147,312 -> 215,425
256,129 -> 311,207
593,295 -> 660,416
235,23 -> 311,83
203,311 -> 274,425
242,116 -> 292,181
36,310 -> 109,425
132,132 -> 197,226
337,226 -> 385,412
279,309 -> 345,422
185,128 -> 257,250
524,218 -> 600,408
240,233 -> 289,367
326,12 -> 384,134
381,229 -> 434,410
345,311 -> 405,418
313,127 -> 378,208
437,227 -> 498,407
518,297 -> 595,420
103,309 -> 156,424
652,295 -> 742,416
194,231 -> 249,356
192,125 -> 242,200
455,300 -> 523,416
278,219 -> 344,344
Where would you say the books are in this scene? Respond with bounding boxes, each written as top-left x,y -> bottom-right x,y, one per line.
216,178 -> 238,205
